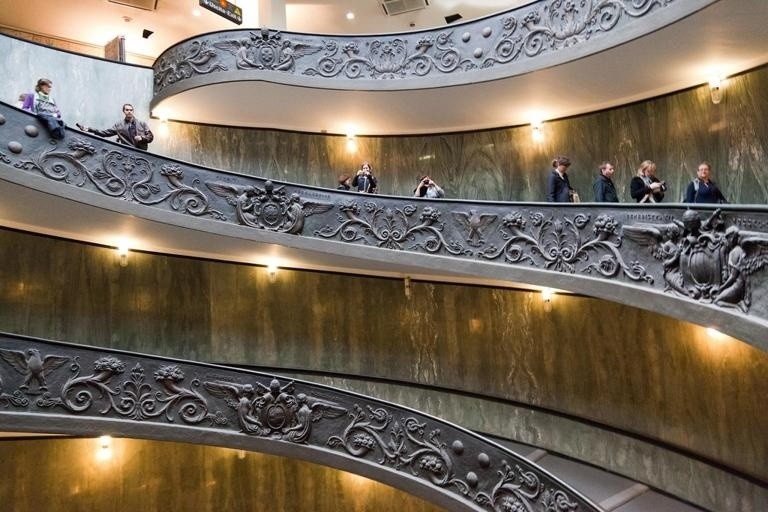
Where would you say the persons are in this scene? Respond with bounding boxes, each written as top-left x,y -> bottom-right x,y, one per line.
74,104 -> 153,151
353,161 -> 377,194
336,173 -> 351,192
593,161 -> 620,204
685,162 -> 728,203
22,78 -> 62,119
630,160 -> 668,203
413,174 -> 445,199
546,157 -> 576,202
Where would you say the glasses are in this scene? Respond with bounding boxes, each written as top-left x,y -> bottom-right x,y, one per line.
126,109 -> 133,111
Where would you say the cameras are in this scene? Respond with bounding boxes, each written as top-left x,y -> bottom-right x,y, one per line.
659,181 -> 667,192
424,180 -> 429,184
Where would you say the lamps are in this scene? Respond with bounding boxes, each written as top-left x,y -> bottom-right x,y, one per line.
99,435 -> 112,450
266,265 -> 277,283
542,288 -> 554,312
118,248 -> 129,269
346,8 -> 354,20
710,79 -> 722,105
531,120 -> 544,144
344,135 -> 359,155
